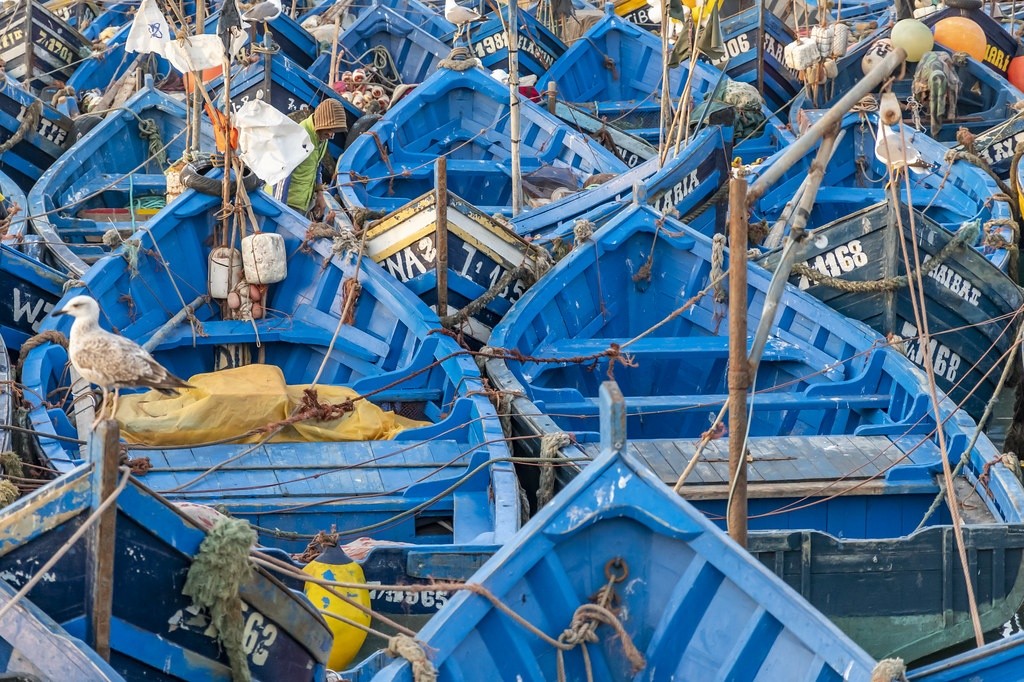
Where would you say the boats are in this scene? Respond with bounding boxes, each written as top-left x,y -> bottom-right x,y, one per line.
0,0 -> 1024,681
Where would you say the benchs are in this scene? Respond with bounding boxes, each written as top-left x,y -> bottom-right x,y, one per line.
386,121 -> 567,180
58,165 -> 167,212
623,128 -> 669,145
760,168 -> 978,220
577,100 -> 695,114
522,330 -> 845,383
356,185 -> 530,218
45,194 -> 146,235
526,349 -> 891,418
131,304 -> 390,362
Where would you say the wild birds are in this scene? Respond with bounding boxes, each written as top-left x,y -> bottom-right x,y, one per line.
443,0 -> 494,45
241,0 -> 284,37
873,115 -> 940,192
50,292 -> 198,432
473,57 -> 485,70
